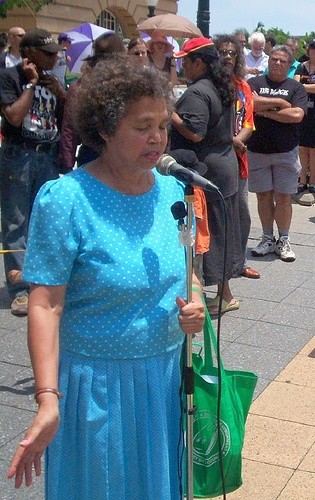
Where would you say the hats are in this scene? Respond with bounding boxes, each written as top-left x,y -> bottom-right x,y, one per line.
58,33 -> 75,41
145,30 -> 174,51
19,29 -> 64,53
169,150 -> 208,177
173,38 -> 214,58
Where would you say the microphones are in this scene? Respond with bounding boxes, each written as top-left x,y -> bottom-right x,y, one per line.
156,154 -> 219,194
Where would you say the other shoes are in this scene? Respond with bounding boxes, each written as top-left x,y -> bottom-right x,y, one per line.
241,268 -> 261,279
11,294 -> 29,316
309,183 -> 315,193
297,183 -> 308,194
205,295 -> 240,314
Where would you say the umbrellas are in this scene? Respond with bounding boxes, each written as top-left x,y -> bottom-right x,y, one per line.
135,13 -> 204,38
57,22 -> 118,75
138,31 -> 182,74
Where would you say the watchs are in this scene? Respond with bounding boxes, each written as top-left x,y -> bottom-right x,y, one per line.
22,83 -> 34,89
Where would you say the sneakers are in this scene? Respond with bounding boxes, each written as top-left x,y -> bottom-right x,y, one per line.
275,235 -> 296,262
251,235 -> 276,257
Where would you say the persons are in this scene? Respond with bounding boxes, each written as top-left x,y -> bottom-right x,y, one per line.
168,38 -> 240,315
7,51 -> 208,500
245,44 -> 308,262
0,26 -> 315,319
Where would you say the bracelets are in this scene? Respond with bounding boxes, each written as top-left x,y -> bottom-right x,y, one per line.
34,388 -> 62,401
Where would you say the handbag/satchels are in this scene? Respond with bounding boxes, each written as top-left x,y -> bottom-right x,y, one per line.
182,288 -> 258,498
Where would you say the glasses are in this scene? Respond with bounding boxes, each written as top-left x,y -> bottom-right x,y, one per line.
219,50 -> 238,58
